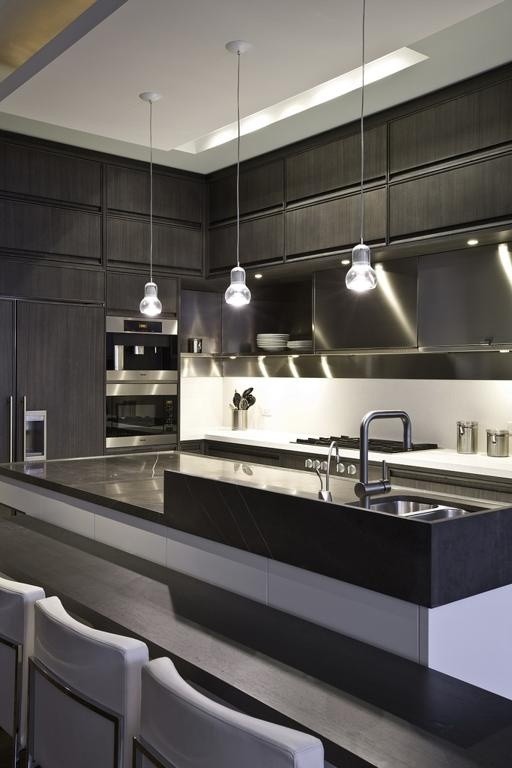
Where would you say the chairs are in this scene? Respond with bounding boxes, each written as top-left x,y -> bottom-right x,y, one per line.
0,570 -> 46,768
132,657 -> 326,768
26,596 -> 150,768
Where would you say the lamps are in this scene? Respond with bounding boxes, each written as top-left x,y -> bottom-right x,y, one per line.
140,93 -> 162,318
225,39 -> 251,309
345,1 -> 376,294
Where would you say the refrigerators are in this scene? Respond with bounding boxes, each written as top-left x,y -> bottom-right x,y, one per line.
0,298 -> 104,462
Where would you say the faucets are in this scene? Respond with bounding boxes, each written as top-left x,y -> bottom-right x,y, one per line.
354,410 -> 412,508
316,441 -> 340,502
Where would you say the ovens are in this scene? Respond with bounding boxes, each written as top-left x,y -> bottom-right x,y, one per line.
106,316 -> 178,447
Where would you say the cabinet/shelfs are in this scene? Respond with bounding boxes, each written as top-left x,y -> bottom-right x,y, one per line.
0,129 -> 105,304
386,76 -> 512,260
103,154 -> 207,319
210,149 -> 287,278
286,116 -> 388,270
180,216 -> 512,378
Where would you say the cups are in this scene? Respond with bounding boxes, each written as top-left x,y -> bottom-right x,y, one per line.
187,338 -> 203,353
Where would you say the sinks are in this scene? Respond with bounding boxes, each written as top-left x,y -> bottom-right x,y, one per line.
409,510 -> 470,520
350,498 -> 438,513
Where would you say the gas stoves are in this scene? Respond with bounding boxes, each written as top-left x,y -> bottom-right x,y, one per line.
294,433 -> 437,454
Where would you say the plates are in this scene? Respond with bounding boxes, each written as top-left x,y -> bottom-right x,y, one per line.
288,340 -> 313,352
255,333 -> 289,353
